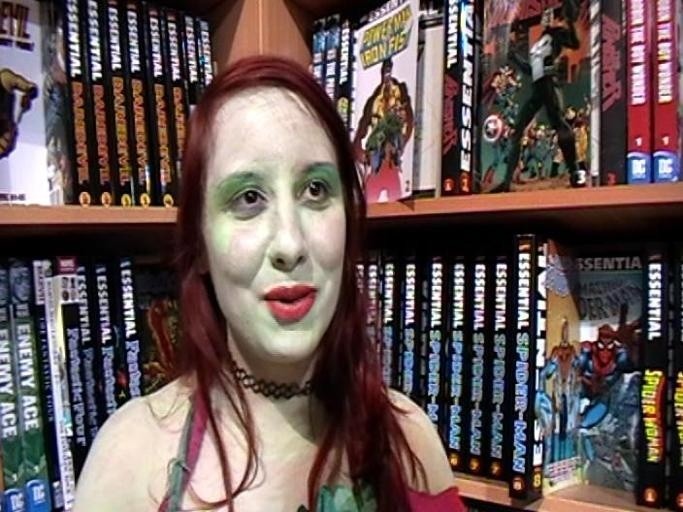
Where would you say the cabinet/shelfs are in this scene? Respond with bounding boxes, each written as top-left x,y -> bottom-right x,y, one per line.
0,2 -> 682,510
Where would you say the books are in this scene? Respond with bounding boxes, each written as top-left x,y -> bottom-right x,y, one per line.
0,0 -> 230,208
0,242 -> 159,512
361,217 -> 683,512
300,0 -> 682,201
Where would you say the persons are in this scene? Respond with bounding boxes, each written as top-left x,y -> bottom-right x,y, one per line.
71,55 -> 467,512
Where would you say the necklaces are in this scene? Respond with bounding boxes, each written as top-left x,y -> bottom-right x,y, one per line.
226,349 -> 325,411
